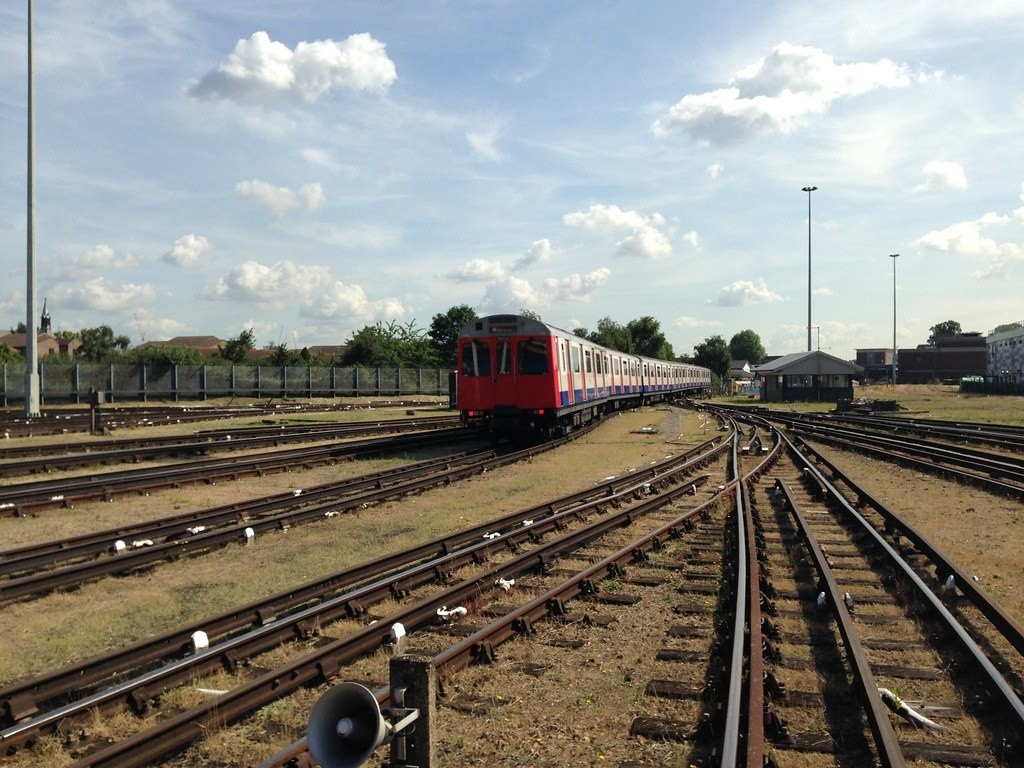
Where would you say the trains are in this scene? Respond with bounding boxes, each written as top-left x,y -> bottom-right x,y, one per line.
455,315 -> 711,442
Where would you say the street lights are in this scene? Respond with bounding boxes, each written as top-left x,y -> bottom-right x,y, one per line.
890,254 -> 900,385
802,185 -> 818,351
